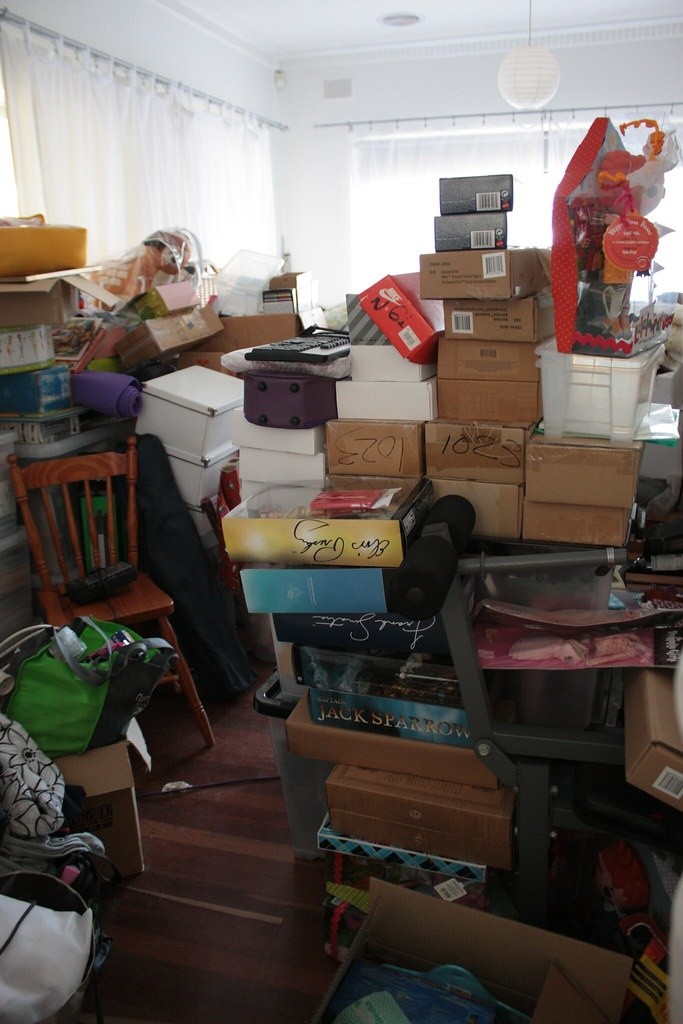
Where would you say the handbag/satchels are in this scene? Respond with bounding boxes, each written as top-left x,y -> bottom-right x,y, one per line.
0,870 -> 114,1024
0,713 -> 67,840
4,615 -> 177,759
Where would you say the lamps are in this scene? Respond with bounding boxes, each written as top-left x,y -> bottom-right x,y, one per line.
496,0 -> 559,109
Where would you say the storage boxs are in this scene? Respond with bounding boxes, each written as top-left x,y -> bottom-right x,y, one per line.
0,175 -> 681,1024
